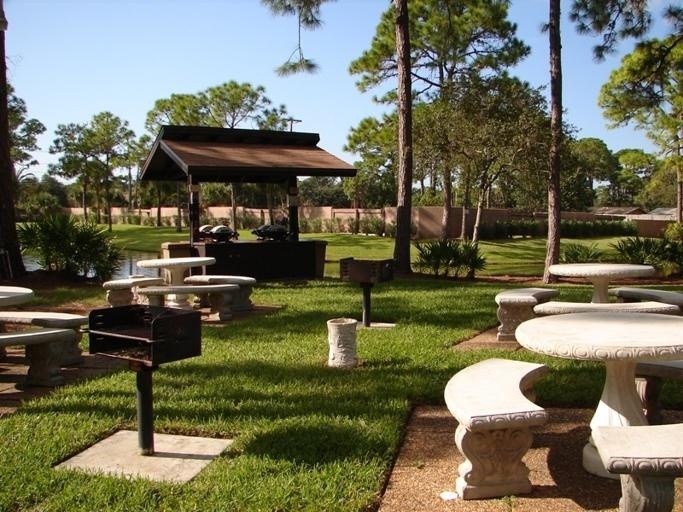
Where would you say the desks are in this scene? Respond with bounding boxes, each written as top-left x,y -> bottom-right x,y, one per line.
135,256 -> 216,311
512,309 -> 682,485
548,263 -> 655,304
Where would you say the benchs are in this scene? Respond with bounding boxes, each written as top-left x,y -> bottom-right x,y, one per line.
0,273 -> 257,388
493,288 -> 561,344
587,418 -> 682,510
531,296 -> 679,318
443,355 -> 552,500
